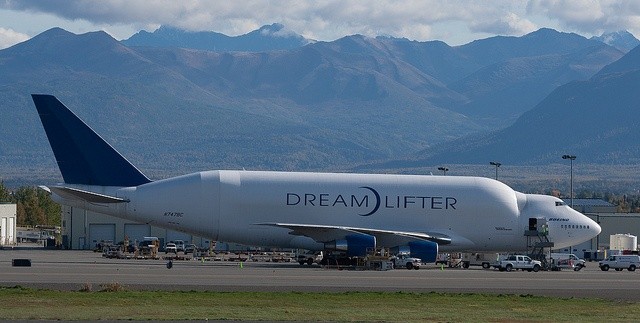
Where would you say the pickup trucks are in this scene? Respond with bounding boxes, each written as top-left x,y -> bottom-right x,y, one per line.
299,248 -> 323,264
494,254 -> 542,271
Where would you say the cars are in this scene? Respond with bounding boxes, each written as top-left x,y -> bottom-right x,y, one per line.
184,243 -> 197,254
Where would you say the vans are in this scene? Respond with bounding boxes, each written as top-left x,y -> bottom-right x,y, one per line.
175,240 -> 184,251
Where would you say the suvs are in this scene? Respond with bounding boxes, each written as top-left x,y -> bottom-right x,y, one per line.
165,242 -> 177,253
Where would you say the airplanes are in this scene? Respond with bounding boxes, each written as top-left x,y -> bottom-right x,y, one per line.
29,92 -> 603,267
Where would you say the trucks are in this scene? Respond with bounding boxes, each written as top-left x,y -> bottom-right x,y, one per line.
598,254 -> 640,271
461,251 -> 491,268
543,252 -> 586,271
390,251 -> 421,269
